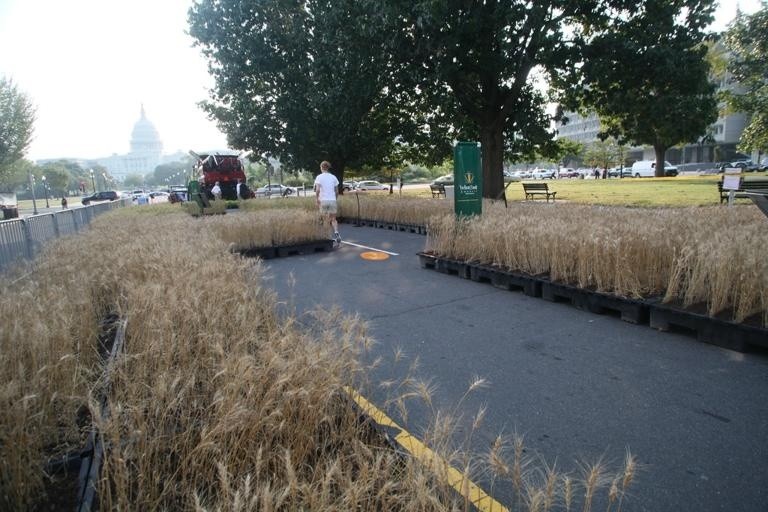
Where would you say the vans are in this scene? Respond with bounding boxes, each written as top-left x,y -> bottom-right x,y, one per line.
631,159 -> 680,179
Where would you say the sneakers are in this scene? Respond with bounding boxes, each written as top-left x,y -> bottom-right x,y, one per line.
334,230 -> 341,243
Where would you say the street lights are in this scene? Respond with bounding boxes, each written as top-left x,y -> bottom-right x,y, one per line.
90,169 -> 96,192
41,175 -> 51,209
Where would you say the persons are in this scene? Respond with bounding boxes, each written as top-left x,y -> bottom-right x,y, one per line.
594,166 -> 601,180
313,160 -> 342,243
549,172 -> 556,180
211,181 -> 223,201
234,178 -> 247,201
602,167 -> 606,179
607,167 -> 610,179
61,197 -> 70,211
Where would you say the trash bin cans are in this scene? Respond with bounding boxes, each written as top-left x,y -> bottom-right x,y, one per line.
444,185 -> 454,198
4,208 -> 18,219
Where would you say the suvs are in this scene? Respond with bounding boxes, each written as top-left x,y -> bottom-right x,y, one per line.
80,190 -> 119,205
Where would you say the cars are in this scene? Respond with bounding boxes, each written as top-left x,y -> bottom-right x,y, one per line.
120,184 -> 192,206
341,179 -> 394,193
501,158 -> 634,180
431,173 -> 454,185
255,182 -> 297,197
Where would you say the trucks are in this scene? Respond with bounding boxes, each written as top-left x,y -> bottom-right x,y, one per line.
190,154 -> 247,201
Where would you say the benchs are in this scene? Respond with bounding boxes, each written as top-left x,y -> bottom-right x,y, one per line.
430,184 -> 446,198
717,181 -> 749,203
522,183 -> 557,203
